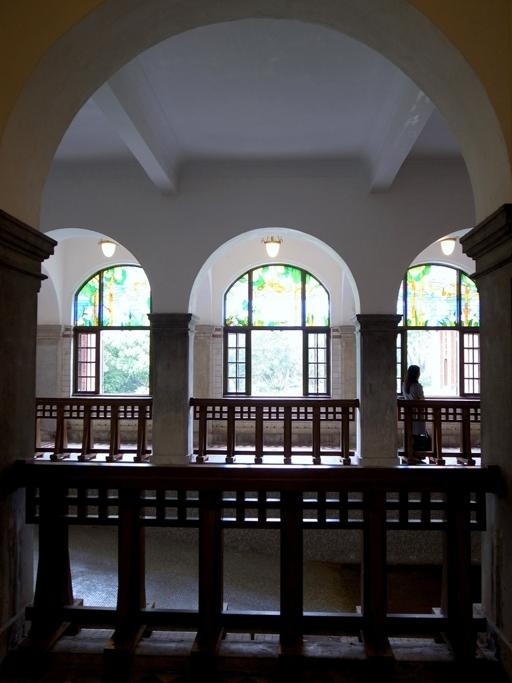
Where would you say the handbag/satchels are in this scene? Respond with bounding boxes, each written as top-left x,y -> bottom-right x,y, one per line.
413,433 -> 432,451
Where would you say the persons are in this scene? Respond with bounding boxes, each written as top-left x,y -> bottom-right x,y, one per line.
402,364 -> 427,462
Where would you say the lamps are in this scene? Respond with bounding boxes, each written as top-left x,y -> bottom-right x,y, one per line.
99,235 -> 118,259
438,235 -> 457,256
260,235 -> 284,258
